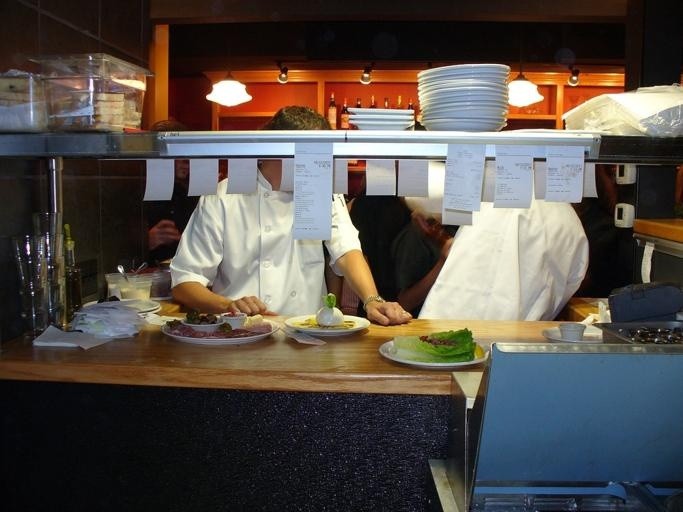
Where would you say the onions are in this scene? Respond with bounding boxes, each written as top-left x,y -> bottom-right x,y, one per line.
316,305 -> 344,327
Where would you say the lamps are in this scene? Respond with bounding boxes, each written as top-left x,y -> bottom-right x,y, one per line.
507,49 -> 544,107
567,66 -> 580,87
360,63 -> 373,84
206,50 -> 253,107
278,61 -> 288,84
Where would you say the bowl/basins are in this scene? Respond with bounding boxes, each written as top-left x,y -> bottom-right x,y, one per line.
106,275 -> 130,298
181,317 -> 225,332
120,276 -> 152,300
222,313 -> 246,329
145,274 -> 170,295
560,323 -> 586,340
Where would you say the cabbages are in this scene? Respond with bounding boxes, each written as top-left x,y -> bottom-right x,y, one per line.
392,327 -> 476,362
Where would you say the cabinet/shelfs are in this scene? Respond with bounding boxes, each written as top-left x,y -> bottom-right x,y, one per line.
204,65 -> 625,196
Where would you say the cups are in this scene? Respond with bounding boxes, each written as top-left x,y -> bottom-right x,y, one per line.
11,209 -> 62,329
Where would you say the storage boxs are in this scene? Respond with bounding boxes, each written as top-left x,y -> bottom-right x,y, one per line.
0,70 -> 48,133
28,53 -> 155,134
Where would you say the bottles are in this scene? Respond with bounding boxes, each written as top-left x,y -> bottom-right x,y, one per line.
382,96 -> 390,106
394,94 -> 405,106
408,98 -> 418,128
339,98 -> 350,126
326,93 -> 338,129
366,92 -> 376,107
354,96 -> 361,105
61,222 -> 83,322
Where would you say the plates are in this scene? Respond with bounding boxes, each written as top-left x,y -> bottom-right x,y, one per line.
379,340 -> 490,369
350,106 -> 416,115
543,328 -> 603,343
353,120 -> 414,129
345,112 -> 413,119
138,306 -> 161,315
161,320 -> 279,344
283,315 -> 370,336
415,63 -> 511,132
85,297 -> 158,311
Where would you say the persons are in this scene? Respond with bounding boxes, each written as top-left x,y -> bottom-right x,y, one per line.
145,106 -> 413,325
328,121 -> 455,322
417,164 -> 636,321
